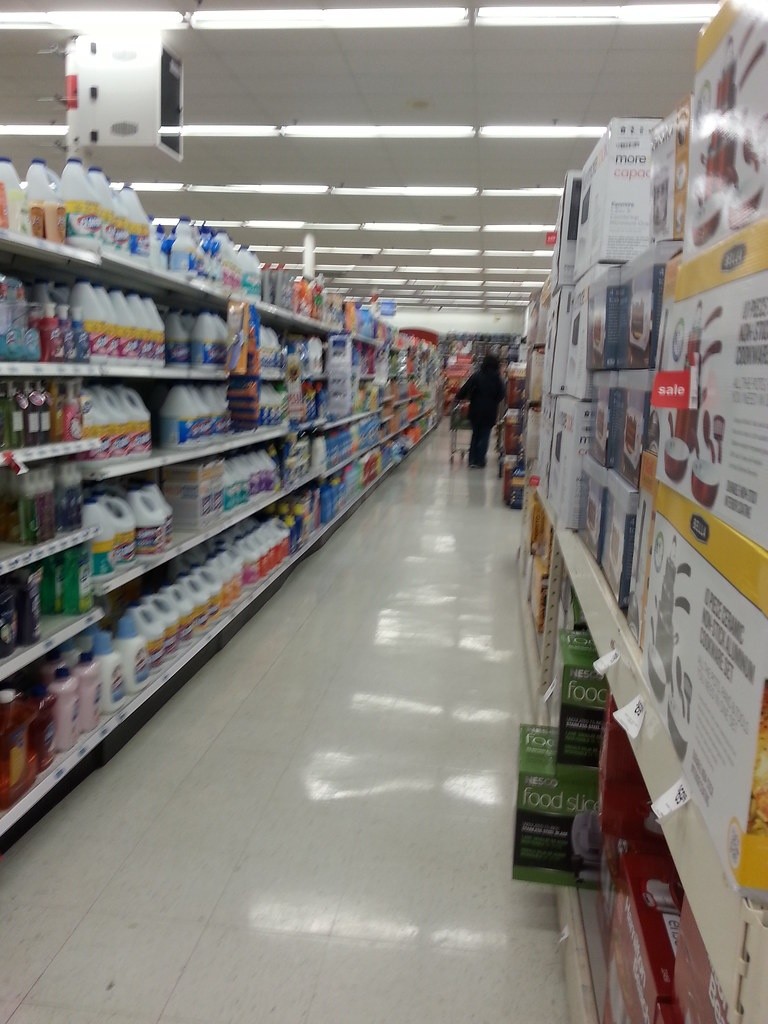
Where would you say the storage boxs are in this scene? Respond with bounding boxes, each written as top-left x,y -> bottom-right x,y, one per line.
502,456 -> 518,505
512,0 -> 768,1024
504,412 -> 524,455
507,377 -> 525,408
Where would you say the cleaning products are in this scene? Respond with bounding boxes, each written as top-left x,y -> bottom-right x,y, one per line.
1,153 -> 420,813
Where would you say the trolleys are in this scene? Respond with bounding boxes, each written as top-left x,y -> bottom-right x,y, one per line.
448,398 -> 474,466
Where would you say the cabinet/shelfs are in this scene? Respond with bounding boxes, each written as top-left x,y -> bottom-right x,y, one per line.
100,240 -> 329,796
0,226 -> 101,858
318,332 -> 440,548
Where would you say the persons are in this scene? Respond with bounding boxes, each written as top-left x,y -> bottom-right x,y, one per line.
455,352 -> 509,469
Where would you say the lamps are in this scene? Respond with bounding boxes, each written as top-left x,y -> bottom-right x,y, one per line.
505,300 -> 530,307
483,280 -> 523,288
152,217 -> 482,233
343,297 -> 508,308
480,186 -> 567,198
247,244 -> 483,257
0,123 -> 475,140
0,6 -> 473,31
323,288 -> 511,298
520,281 -> 544,288
312,278 -> 484,287
472,0 -> 727,24
509,291 -> 531,297
481,224 -> 557,232
482,249 -> 555,258
258,262 -> 483,274
20,181 -> 479,197
483,268 -> 552,275
477,125 -> 607,139
